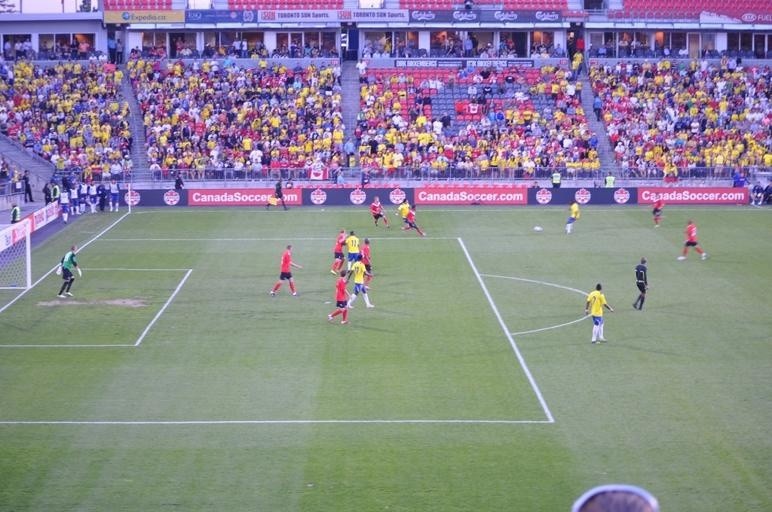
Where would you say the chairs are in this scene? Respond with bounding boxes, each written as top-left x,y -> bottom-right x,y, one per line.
0,38 -> 772,195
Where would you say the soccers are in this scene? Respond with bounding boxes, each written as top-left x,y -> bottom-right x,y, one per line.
534,227 -> 543,233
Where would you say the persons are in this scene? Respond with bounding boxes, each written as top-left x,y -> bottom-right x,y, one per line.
1,33 -> 772,238
270,245 -> 305,299
327,228 -> 376,326
56,244 -> 84,301
569,482 -> 661,511
632,256 -> 650,311
584,283 -> 615,344
678,220 -> 708,262
